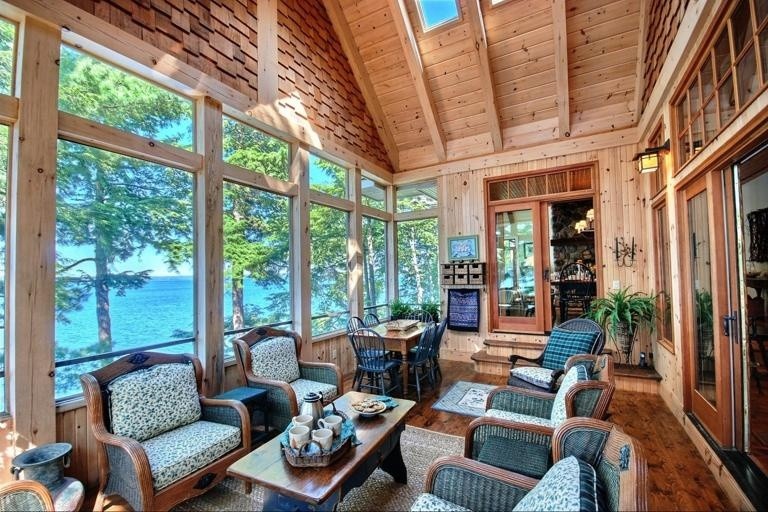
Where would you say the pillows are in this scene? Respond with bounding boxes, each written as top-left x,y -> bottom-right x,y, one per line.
542,325 -> 601,372
509,365 -> 566,390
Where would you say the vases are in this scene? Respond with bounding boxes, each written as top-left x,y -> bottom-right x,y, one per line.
9,443 -> 83,512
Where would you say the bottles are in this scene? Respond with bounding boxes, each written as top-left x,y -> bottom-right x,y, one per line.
576,259 -> 584,279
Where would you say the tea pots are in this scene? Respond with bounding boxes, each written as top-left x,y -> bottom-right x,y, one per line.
298,390 -> 325,427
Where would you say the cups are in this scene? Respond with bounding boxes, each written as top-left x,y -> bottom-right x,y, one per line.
288,426 -> 310,449
317,415 -> 343,436
291,415 -> 313,430
567,271 -> 592,282
310,427 -> 332,453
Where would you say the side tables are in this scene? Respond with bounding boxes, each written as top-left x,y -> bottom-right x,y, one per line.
212,387 -> 268,445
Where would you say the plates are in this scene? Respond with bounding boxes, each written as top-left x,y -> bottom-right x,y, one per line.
348,400 -> 386,418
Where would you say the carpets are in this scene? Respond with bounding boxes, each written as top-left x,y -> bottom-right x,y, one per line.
166,423 -> 464,510
430,379 -> 498,417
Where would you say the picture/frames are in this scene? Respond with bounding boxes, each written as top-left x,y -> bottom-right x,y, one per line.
447,234 -> 479,261
523,242 -> 533,258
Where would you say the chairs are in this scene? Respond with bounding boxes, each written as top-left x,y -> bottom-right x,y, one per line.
344,310 -> 449,397
409,417 -> 648,510
230,326 -> 344,433
498,290 -> 534,318
560,263 -> 593,324
0,480 -> 54,512
78,350 -> 251,512
464,354 -> 615,479
507,319 -> 605,392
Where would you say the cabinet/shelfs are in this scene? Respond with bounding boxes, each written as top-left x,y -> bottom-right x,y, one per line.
440,262 -> 485,286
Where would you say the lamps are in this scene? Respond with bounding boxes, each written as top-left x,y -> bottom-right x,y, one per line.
632,139 -> 669,174
575,209 -> 594,233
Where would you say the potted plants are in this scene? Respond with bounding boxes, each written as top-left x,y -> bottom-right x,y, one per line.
661,290 -> 713,361
583,283 -> 660,370
